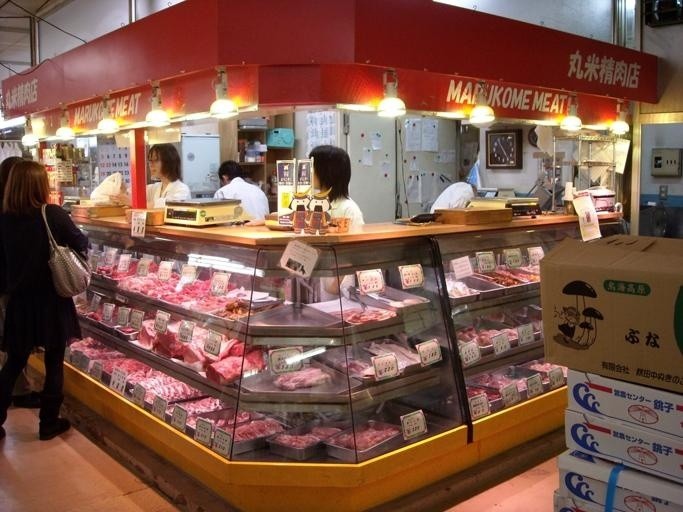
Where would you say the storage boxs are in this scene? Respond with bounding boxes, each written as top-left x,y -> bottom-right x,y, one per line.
551,489 -> 609,511
552,405 -> 680,485
527,229 -> 682,390
556,449 -> 680,510
559,362 -> 680,439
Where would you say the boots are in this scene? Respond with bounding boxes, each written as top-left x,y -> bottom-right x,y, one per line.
38,391 -> 71,440
0,395 -> 12,440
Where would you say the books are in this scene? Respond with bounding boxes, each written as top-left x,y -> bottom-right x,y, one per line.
434,207 -> 514,224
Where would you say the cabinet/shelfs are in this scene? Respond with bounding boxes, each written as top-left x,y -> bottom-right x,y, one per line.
217,109 -> 295,198
30,203 -> 626,512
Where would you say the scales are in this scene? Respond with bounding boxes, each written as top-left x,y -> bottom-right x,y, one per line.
164,198 -> 245,227
476,187 -> 515,198
61,196 -> 80,213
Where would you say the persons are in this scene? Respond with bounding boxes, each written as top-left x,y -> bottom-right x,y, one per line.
429,163 -> 480,217
212,159 -> 269,225
263,144 -> 365,227
0,159 -> 89,441
0,155 -> 23,210
105,143 -> 191,207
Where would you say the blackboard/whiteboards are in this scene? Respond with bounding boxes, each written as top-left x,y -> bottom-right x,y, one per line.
181,135 -> 221,194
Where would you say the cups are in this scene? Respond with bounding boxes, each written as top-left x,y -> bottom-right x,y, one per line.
563,199 -> 574,215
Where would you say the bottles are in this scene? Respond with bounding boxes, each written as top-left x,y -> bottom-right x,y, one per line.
270,168 -> 277,194
47,191 -> 62,207
238,137 -> 264,162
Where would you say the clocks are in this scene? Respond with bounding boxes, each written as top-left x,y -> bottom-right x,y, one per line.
484,130 -> 521,170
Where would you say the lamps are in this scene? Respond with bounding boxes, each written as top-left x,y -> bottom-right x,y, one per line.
607,99 -> 632,136
204,64 -> 240,121
96,95 -> 117,132
143,79 -> 169,129
18,112 -> 39,148
377,67 -> 408,120
56,106 -> 74,144
559,92 -> 583,135
468,79 -> 495,123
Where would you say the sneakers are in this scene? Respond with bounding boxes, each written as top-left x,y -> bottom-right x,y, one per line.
11,390 -> 45,410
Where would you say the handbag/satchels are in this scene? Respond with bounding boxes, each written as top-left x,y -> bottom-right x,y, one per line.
48,244 -> 92,298
